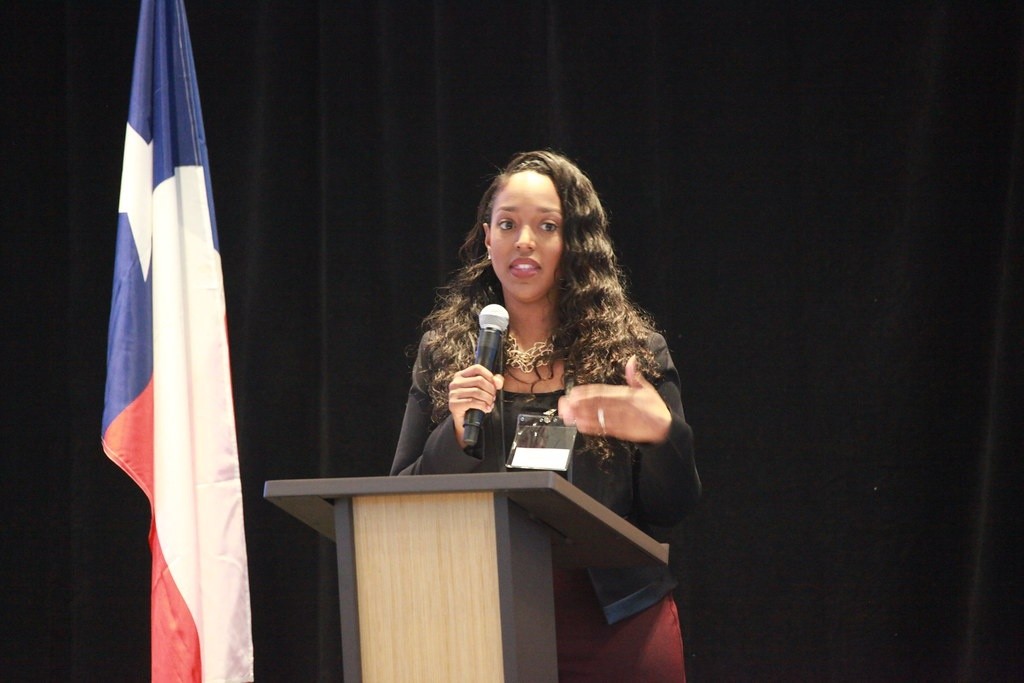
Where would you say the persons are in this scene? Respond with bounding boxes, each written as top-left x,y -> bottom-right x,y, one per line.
392,151 -> 701,683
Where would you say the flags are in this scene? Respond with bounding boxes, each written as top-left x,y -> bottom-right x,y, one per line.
101,1 -> 254,683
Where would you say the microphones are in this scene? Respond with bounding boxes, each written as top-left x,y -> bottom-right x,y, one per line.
462,304 -> 511,446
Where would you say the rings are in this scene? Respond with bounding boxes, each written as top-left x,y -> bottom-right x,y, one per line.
598,409 -> 606,427
